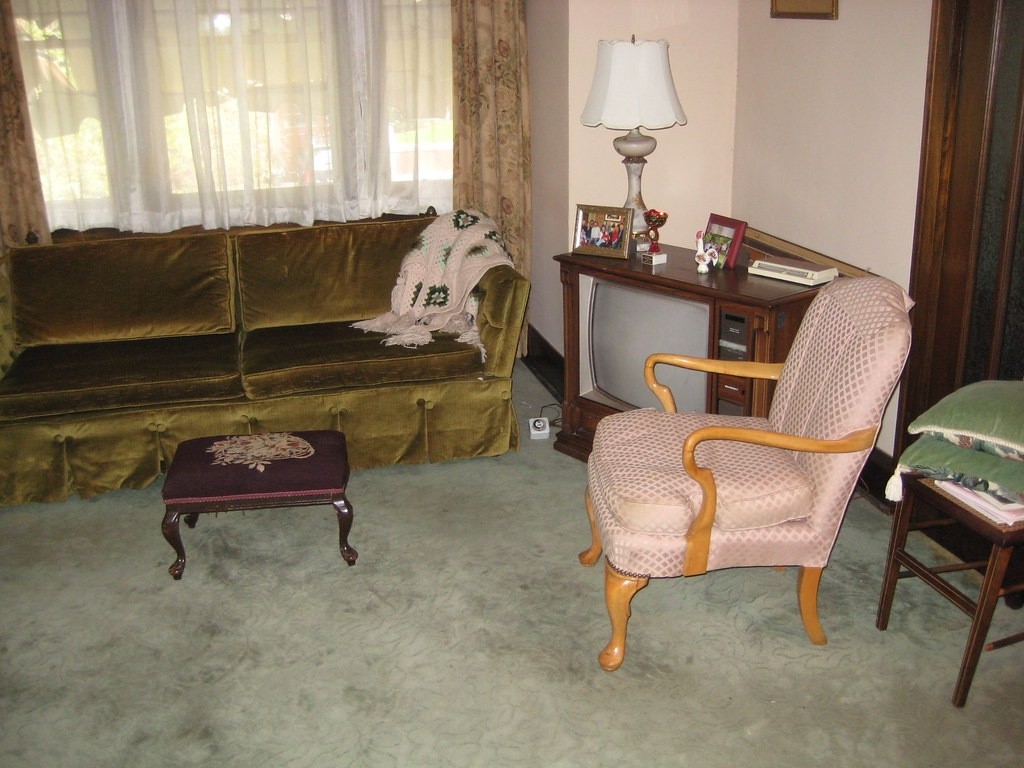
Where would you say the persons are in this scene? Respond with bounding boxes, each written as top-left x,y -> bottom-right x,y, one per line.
703,236 -> 722,261
580,219 -> 624,249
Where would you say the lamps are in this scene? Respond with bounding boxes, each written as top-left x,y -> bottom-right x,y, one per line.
581,31 -> 689,252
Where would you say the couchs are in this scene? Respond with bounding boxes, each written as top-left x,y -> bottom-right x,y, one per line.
1,206 -> 532,508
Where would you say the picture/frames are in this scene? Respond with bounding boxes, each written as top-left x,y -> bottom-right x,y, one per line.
572,202 -> 635,259
706,212 -> 749,269
770,0 -> 838,19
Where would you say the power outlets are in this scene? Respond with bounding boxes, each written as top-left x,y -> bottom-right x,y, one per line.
528,415 -> 549,440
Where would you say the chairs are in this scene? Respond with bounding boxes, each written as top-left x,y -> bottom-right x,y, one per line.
578,272 -> 913,674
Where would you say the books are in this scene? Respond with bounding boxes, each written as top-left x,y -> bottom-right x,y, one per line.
934,475 -> 1024,527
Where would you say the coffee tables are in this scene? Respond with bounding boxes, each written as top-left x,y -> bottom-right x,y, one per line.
160,430 -> 358,581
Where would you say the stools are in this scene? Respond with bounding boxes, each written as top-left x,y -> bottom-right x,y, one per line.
875,472 -> 1024,708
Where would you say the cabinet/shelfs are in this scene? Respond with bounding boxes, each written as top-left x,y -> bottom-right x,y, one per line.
553,239 -> 827,465
